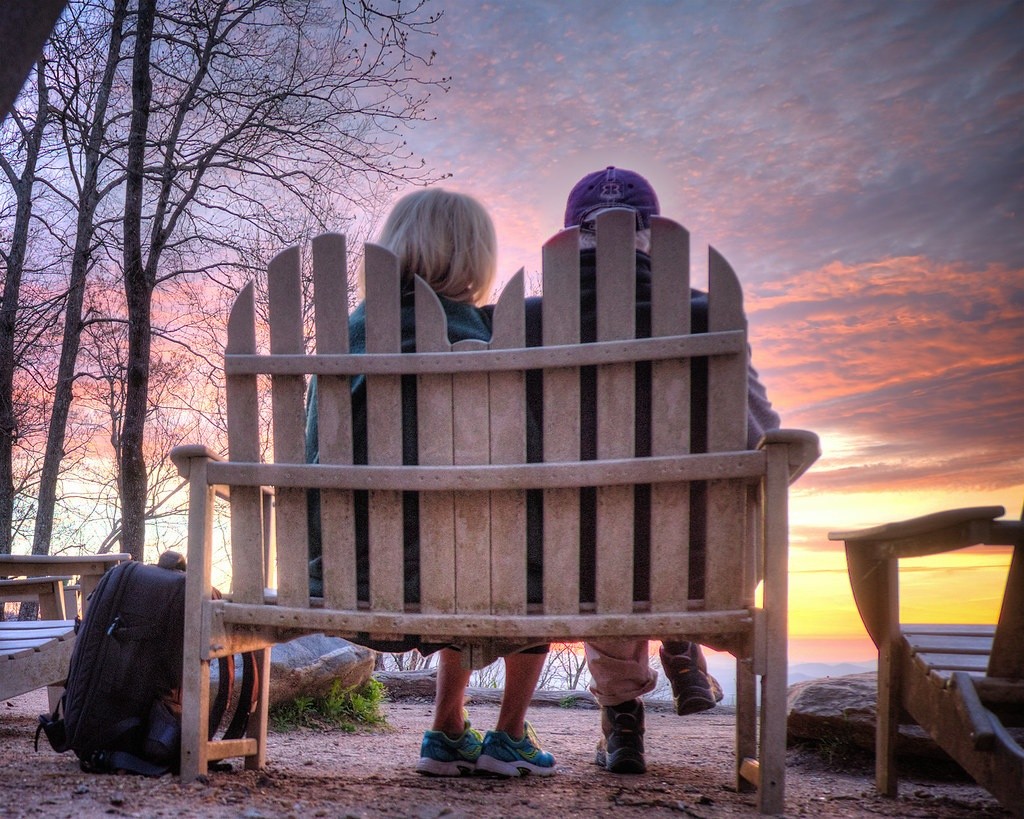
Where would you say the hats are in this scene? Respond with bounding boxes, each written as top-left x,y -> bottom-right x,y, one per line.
564,166 -> 660,234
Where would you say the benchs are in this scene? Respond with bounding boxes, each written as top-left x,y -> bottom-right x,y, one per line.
168,207 -> 822,819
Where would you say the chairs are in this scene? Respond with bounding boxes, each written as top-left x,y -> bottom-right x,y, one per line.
828,505 -> 1024,819
0,553 -> 133,716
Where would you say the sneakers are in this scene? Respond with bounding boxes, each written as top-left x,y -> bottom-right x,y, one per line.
416,708 -> 484,777
659,643 -> 724,716
594,699 -> 647,774
476,721 -> 558,776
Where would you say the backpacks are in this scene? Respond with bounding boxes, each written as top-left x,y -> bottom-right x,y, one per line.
34,551 -> 258,779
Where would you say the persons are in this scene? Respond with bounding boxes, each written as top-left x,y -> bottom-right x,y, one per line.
292,187 -> 559,776
474,163 -> 782,773
